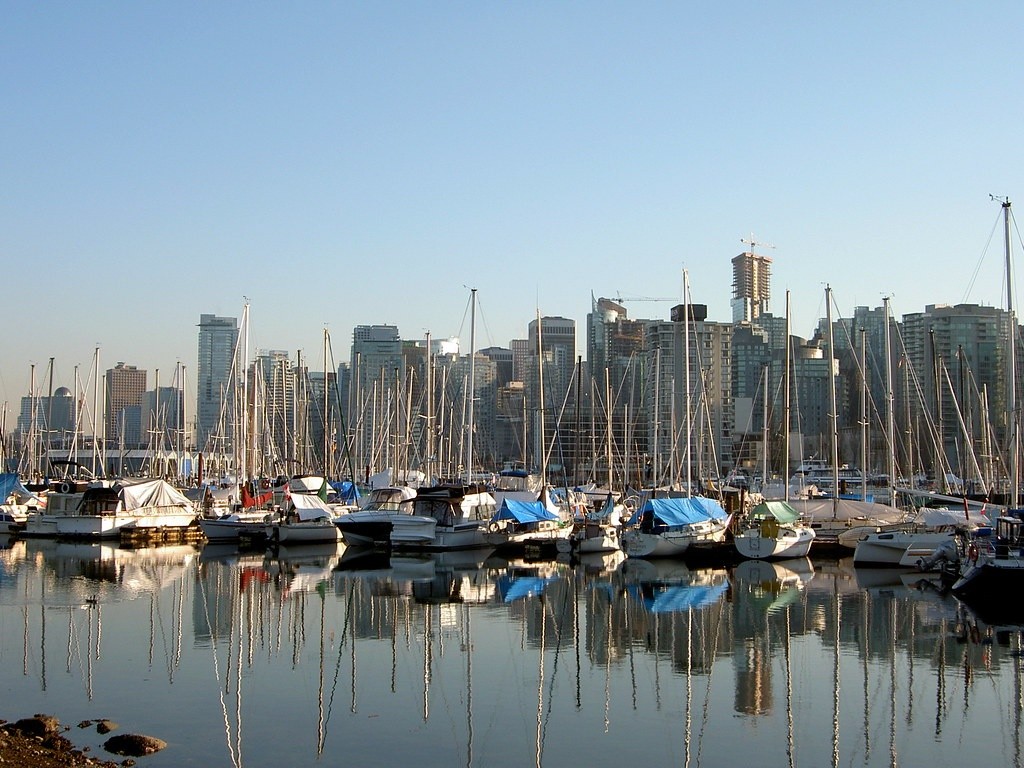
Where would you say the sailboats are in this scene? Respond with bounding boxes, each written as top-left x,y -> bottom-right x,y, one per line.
0,195 -> 1024,626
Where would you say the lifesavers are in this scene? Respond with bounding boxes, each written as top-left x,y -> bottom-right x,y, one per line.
263,515 -> 272,525
61,483 -> 69,493
489,522 -> 499,533
969,545 -> 978,561
262,480 -> 269,490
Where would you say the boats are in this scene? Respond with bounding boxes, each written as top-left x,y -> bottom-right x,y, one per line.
94,549 -> 816,614
